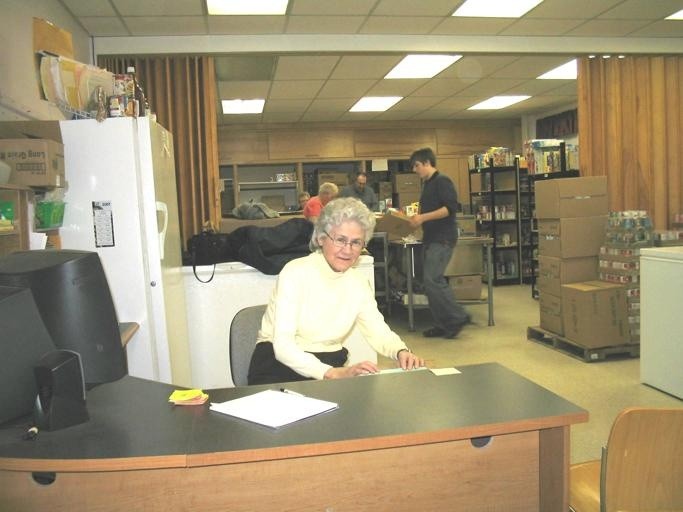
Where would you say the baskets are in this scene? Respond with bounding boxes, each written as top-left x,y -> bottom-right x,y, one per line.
34,201 -> 65,229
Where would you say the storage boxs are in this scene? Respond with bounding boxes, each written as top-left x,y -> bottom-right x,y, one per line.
448,276 -> 482,300
535,176 -> 630,348
1,120 -> 65,186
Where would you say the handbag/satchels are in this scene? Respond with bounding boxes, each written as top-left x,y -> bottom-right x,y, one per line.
187,231 -> 234,266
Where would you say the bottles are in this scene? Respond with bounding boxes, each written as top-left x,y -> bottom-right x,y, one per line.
137,85 -> 144,116
121,66 -> 139,116
145,98 -> 150,117
96,87 -> 109,119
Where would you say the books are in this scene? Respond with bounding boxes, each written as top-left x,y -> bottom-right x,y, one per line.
209,389 -> 341,433
492,232 -> 518,246
521,202 -> 536,219
518,138 -> 579,177
473,204 -> 492,222
377,211 -> 418,237
492,249 -> 519,281
522,244 -> 539,284
468,146 -> 516,169
492,204 -> 516,221
520,219 -> 538,244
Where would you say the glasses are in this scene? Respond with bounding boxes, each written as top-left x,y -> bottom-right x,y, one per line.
322,230 -> 367,251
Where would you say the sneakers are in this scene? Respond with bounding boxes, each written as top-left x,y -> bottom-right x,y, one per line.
423,328 -> 445,337
445,317 -> 470,339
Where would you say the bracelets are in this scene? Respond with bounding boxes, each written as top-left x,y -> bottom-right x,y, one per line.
395,348 -> 412,358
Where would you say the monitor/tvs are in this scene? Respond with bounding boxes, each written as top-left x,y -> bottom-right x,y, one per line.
0,248 -> 128,424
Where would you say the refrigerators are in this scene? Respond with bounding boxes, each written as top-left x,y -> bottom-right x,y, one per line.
47,116 -> 195,385
638,247 -> 682,403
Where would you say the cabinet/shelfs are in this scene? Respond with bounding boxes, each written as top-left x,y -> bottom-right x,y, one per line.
467,143 -> 539,298
368,231 -> 390,315
528,171 -> 578,297
219,156 -> 470,218
0,187 -> 58,253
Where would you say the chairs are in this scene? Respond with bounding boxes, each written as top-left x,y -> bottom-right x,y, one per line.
229,304 -> 267,387
569,406 -> 683,512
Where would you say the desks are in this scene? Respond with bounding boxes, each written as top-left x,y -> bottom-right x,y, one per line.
392,237 -> 495,332
0,322 -> 590,511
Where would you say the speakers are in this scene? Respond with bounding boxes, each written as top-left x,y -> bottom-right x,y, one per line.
33,350 -> 89,432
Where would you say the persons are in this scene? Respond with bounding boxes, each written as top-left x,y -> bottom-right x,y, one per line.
408,146 -> 472,339
340,171 -> 379,213
303,182 -> 339,225
246,196 -> 426,385
295,192 -> 312,211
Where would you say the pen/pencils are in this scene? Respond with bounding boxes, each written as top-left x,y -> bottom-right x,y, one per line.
280,388 -> 306,397
